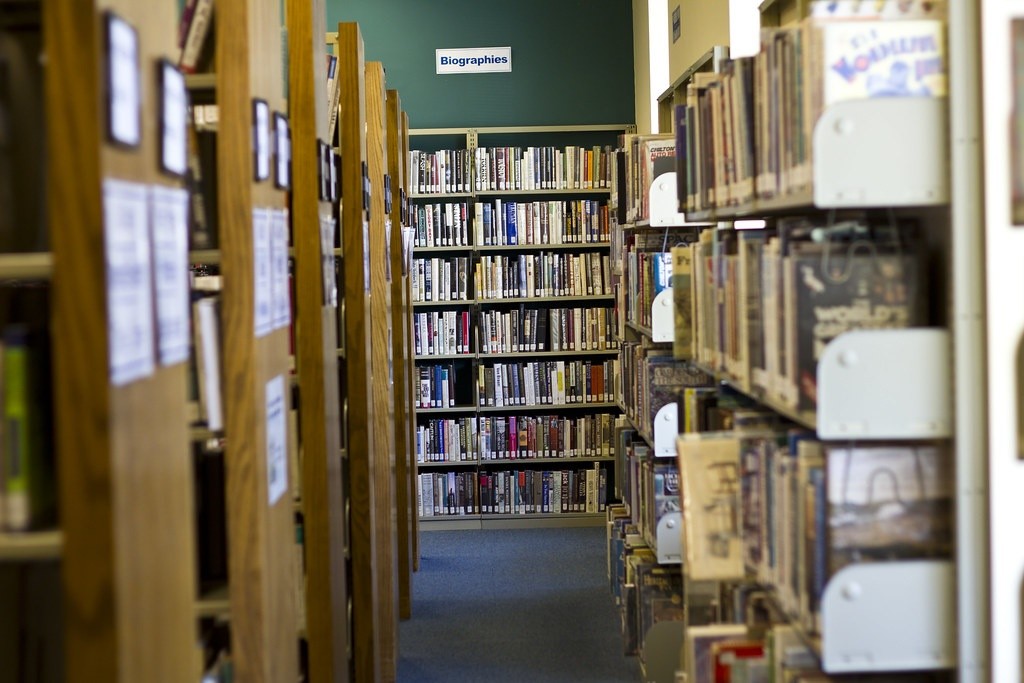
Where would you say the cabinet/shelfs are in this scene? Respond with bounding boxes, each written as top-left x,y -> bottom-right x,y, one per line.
407,123 -> 637,531
617,0 -> 989,683
0,0 -> 413,683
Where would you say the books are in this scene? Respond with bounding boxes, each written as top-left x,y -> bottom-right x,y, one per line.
0,0 -> 67,682
415,361 -> 624,408
411,252 -> 612,304
666,226 -> 918,412
418,462 -> 607,516
672,383 -> 960,683
409,145 -> 611,193
418,414 -> 625,462
417,198 -> 614,247
176,1 -> 230,600
275,23 -> 359,682
669,14 -> 949,214
607,131 -> 694,671
415,306 -> 617,356
